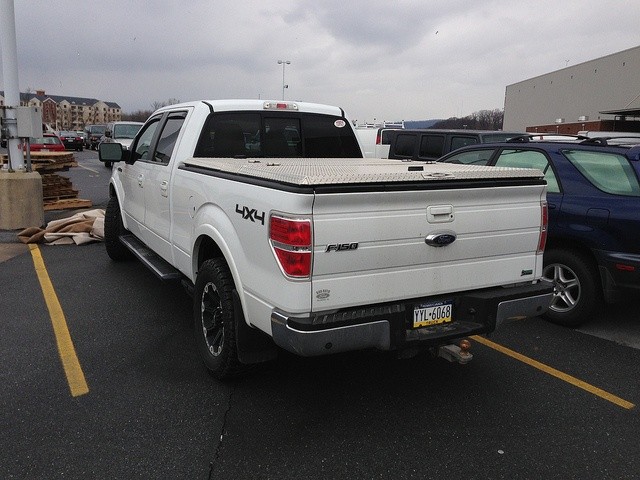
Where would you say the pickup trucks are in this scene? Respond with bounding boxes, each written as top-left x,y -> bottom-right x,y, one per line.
349,119 -> 406,160
96,97 -> 559,382
84,125 -> 109,150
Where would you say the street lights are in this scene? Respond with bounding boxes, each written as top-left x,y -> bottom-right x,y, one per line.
276,59 -> 292,101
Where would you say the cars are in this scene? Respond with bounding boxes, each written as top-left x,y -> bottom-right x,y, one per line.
419,142 -> 640,330
53,131 -> 83,152
23,134 -> 65,152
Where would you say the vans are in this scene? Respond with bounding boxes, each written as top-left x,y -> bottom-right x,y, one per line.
102,121 -> 146,167
388,129 -> 534,162
